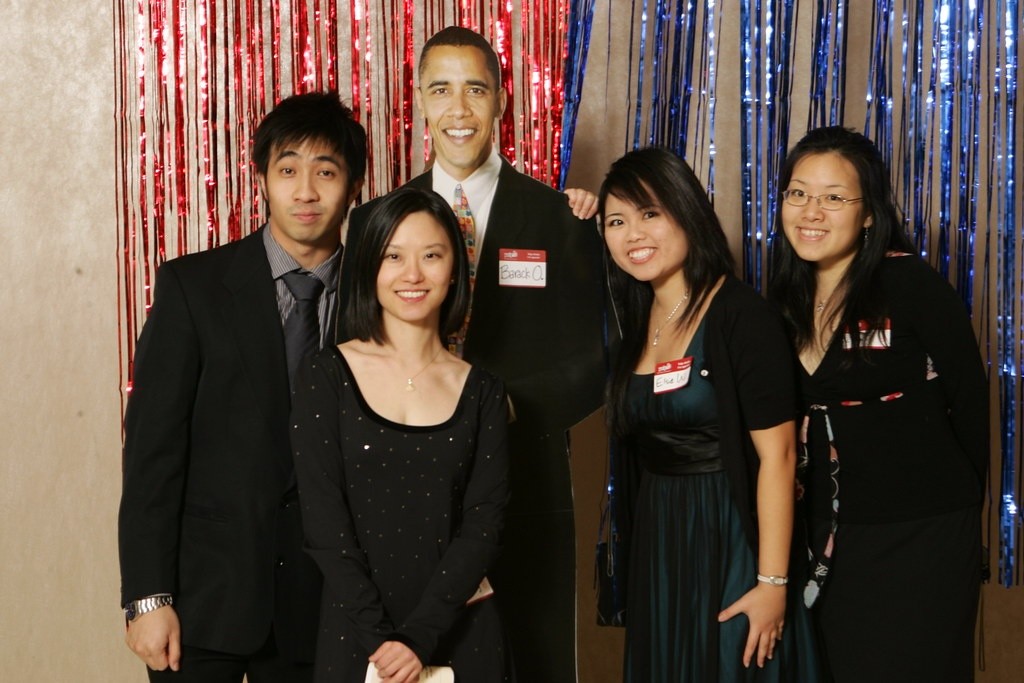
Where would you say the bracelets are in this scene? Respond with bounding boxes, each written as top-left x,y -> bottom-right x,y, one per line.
758,574 -> 789,586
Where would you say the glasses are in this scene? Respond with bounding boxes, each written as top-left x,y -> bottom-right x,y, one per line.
781,189 -> 864,211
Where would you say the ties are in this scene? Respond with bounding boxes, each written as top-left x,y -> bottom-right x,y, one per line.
281,270 -> 325,412
447,184 -> 477,362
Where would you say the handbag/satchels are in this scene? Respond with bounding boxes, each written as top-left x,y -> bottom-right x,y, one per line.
594,539 -> 628,627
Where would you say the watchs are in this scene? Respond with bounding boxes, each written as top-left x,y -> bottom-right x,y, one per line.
126,595 -> 173,620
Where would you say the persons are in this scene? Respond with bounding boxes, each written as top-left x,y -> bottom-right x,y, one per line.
287,186 -> 511,683
119,93 -> 371,682
325,26 -> 609,682
766,126 -> 992,683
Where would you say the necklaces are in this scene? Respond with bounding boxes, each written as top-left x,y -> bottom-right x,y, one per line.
563,147 -> 821,683
815,301 -> 825,313
406,345 -> 444,393
642,293 -> 692,347
